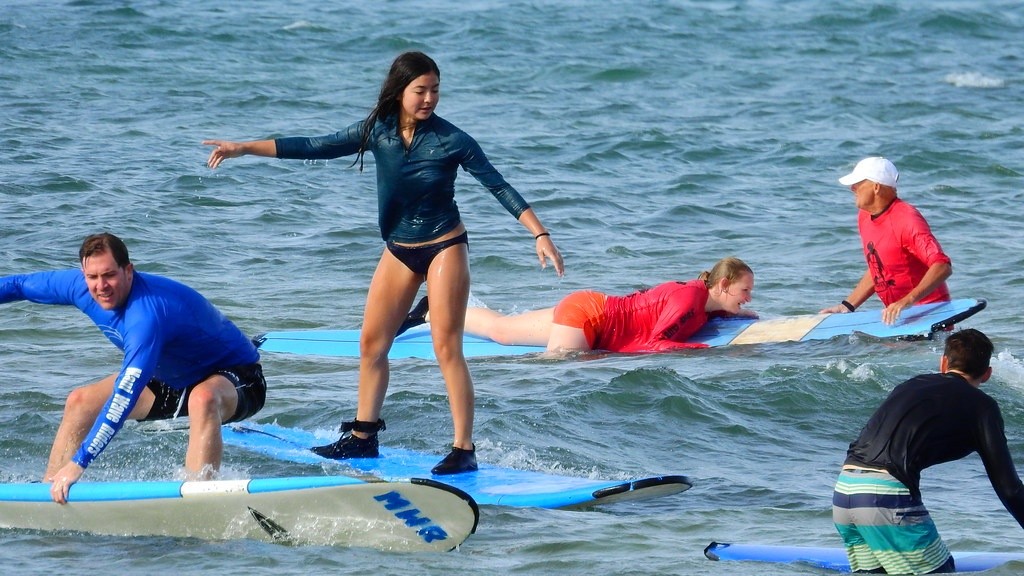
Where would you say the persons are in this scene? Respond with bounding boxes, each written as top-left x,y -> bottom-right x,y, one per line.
199,50 -> 568,479
817,154 -> 955,342
395,254 -> 759,353
828,326 -> 1024,575
0,230 -> 269,504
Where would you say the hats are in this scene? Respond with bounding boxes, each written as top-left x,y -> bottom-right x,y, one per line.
838,157 -> 899,187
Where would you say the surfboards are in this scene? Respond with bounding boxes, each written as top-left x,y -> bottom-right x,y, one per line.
705,539 -> 1023,576
0,477 -> 478,556
220,422 -> 693,510
251,296 -> 988,360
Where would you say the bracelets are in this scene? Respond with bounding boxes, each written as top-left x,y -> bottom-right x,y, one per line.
533,232 -> 553,242
841,300 -> 854,313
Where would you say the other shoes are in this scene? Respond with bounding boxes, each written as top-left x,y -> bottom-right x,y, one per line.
395,295 -> 430,337
430,443 -> 479,476
310,436 -> 379,461
